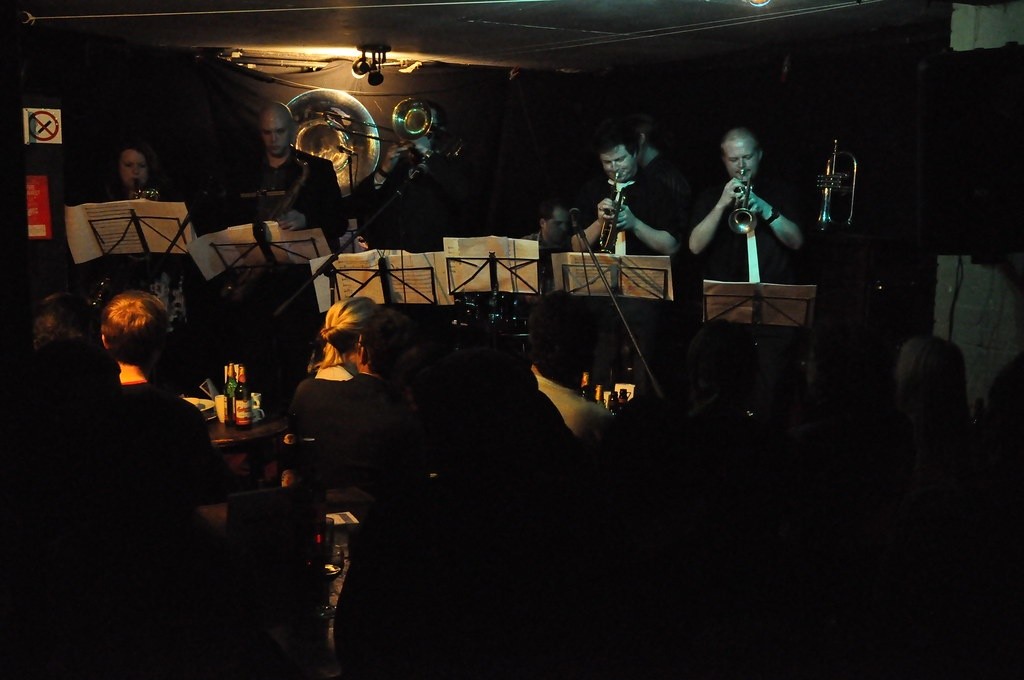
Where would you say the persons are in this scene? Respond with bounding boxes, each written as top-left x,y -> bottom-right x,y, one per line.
0,102 -> 1024,680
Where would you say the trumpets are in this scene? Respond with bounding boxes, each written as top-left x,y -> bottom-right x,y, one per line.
595,174 -> 626,254
729,168 -> 758,236
809,138 -> 858,232
391,96 -> 454,142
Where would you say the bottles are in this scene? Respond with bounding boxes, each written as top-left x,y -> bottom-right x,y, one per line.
235,363 -> 253,430
224,362 -> 238,426
579,371 -> 627,415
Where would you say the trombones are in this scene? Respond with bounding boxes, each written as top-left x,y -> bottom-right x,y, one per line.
322,109 -> 406,145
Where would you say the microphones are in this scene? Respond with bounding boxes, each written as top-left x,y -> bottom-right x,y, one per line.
336,144 -> 355,156
569,207 -> 582,229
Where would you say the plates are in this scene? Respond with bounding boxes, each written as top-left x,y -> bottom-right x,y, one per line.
183,398 -> 214,411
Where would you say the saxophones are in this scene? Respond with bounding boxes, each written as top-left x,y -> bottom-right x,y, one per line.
219,154 -> 311,301
85,178 -> 161,309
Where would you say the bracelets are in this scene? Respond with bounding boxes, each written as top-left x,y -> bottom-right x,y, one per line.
767,208 -> 779,224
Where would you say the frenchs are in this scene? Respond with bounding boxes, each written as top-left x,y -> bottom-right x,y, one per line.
285,87 -> 382,199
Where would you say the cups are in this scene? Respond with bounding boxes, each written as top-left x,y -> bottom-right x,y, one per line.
215,395 -> 225,422
251,392 -> 261,409
